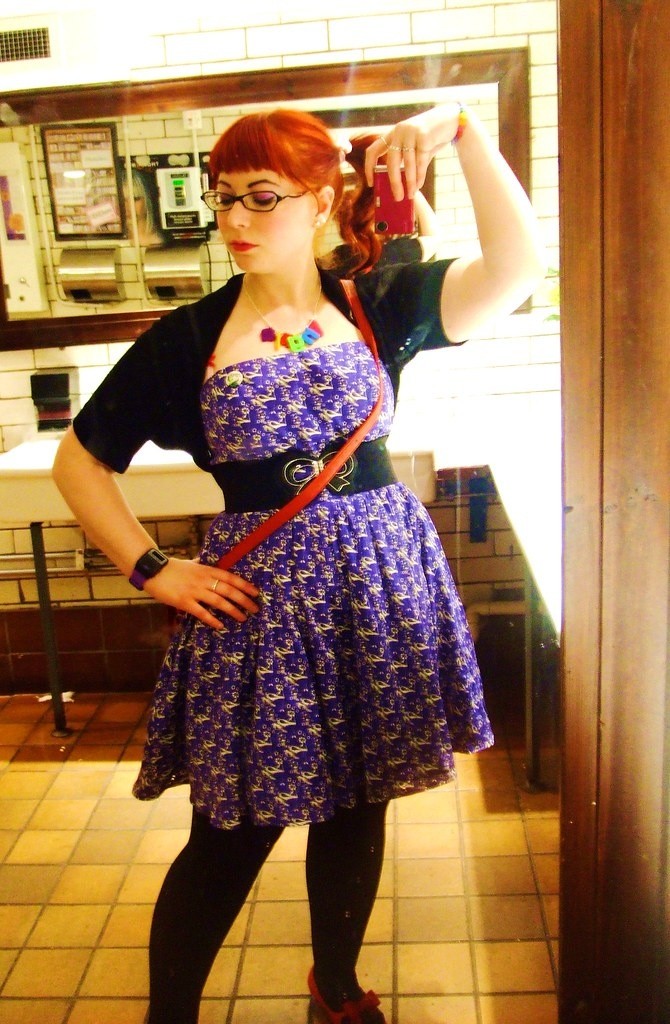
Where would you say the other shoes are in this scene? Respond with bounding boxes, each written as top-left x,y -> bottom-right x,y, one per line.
308,963 -> 386,1024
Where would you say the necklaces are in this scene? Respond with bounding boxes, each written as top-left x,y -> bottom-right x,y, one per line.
242,274 -> 325,352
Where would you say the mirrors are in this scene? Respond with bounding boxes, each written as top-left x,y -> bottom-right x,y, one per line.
0,45 -> 532,352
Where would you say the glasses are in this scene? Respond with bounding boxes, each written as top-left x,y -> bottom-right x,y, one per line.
200,188 -> 311,212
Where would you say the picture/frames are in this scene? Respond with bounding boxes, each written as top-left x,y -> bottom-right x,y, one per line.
40,121 -> 127,242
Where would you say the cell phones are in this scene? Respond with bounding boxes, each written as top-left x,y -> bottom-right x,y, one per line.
374,164 -> 415,234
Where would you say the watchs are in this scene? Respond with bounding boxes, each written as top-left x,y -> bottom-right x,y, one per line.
129,549 -> 167,591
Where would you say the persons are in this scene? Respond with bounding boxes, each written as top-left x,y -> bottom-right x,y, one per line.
50,98 -> 546,1024
375,189 -> 443,265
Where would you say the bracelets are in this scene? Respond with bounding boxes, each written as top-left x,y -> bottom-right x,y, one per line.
450,101 -> 467,146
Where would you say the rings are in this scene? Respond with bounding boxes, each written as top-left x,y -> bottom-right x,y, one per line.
381,138 -> 389,148
403,146 -> 417,151
212,579 -> 220,590
388,146 -> 399,151
